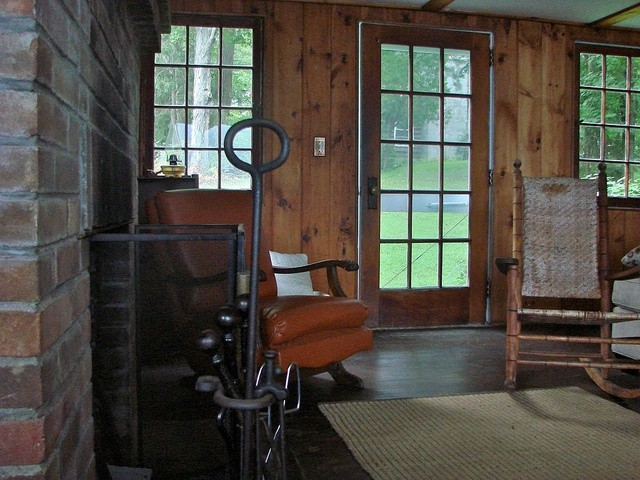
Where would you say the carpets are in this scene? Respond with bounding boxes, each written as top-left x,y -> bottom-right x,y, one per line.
316,386 -> 640,480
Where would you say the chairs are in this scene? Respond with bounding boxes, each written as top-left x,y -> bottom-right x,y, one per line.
494,160 -> 638,400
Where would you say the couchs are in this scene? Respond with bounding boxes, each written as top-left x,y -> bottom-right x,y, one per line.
145,188 -> 368,398
